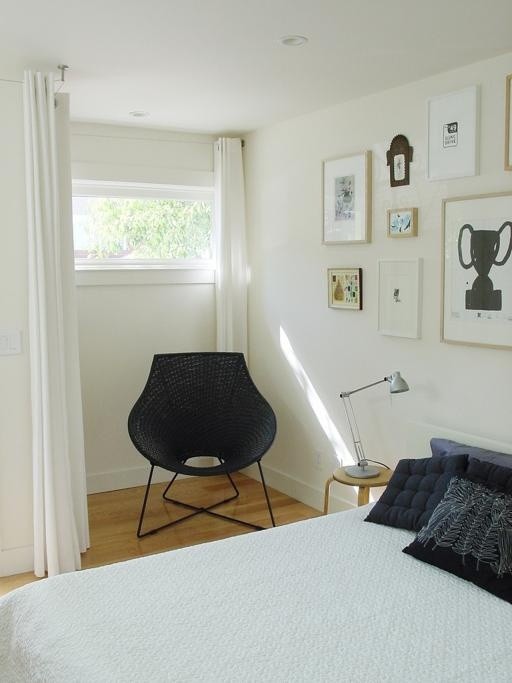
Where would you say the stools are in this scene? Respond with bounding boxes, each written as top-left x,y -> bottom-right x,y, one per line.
324,465 -> 394,515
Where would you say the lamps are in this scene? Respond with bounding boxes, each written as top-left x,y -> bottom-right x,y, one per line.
339,371 -> 409,479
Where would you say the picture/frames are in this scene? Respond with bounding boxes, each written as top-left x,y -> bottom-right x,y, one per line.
377,257 -> 422,339
387,208 -> 418,238
321,150 -> 371,246
440,191 -> 512,350
386,134 -> 413,187
426,86 -> 480,181
327,267 -> 362,310
504,74 -> 512,171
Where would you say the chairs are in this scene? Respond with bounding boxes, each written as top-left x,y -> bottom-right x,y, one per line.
127,352 -> 277,537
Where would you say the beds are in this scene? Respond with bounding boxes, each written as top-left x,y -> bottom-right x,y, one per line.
0,423 -> 512,683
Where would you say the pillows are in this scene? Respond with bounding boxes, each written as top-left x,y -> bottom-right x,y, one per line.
466,456 -> 512,494
430,437 -> 511,468
402,472 -> 512,605
364,454 -> 468,530
